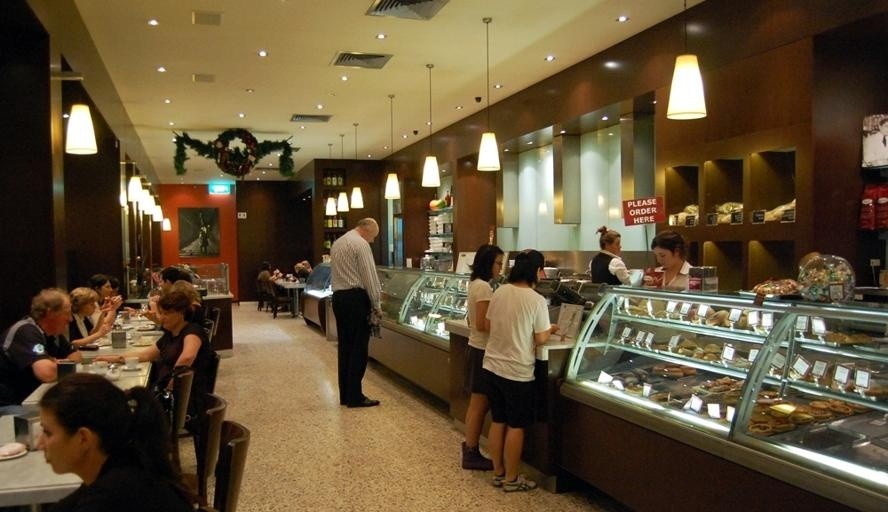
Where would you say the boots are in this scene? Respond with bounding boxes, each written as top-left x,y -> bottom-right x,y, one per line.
462,441 -> 493,471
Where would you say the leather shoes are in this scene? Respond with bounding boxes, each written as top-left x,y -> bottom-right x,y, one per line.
340,397 -> 381,408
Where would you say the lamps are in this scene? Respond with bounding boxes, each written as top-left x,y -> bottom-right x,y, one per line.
337,133 -> 350,212
666,0 -> 711,123
476,16 -> 503,173
420,64 -> 442,188
126,162 -> 172,231
64,102 -> 99,157
350,121 -> 365,209
326,144 -> 337,216
383,93 -> 402,200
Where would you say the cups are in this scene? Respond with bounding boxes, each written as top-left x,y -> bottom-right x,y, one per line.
141,303 -> 150,312
111,331 -> 126,348
124,357 -> 139,368
629,269 -> 644,287
406,258 -> 412,267
542,266 -> 559,280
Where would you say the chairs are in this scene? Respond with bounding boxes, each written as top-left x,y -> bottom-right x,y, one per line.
256,276 -> 306,319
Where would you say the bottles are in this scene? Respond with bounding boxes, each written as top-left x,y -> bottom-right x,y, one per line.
323,171 -> 345,250
106,364 -> 120,381
444,190 -> 454,207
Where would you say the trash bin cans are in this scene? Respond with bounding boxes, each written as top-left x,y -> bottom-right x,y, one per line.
326,295 -> 338,341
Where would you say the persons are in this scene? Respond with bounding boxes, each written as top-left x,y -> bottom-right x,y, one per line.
330,218 -> 383,407
486,249 -> 560,492
589,226 -> 632,285
0,267 -> 217,411
461,244 -> 503,469
651,231 -> 694,290
37,371 -> 195,510
257,260 -> 313,312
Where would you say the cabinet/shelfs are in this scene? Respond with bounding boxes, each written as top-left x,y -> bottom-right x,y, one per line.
563,285 -> 888,511
399,271 -> 506,342
652,149 -> 861,303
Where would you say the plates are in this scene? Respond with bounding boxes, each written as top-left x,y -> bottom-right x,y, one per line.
0,449 -> 27,460
122,364 -> 143,371
135,324 -> 155,331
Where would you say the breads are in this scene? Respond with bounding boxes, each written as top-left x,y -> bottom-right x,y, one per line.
615,322 -> 751,370
822,330 -> 872,345
626,298 -> 754,330
594,362 -> 747,428
768,348 -> 888,399
747,388 -> 869,439
711,405 -> 719,406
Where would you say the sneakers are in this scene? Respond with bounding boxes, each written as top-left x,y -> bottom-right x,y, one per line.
493,472 -> 537,492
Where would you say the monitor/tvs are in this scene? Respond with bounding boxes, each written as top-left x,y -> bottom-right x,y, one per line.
454,252 -> 476,274
